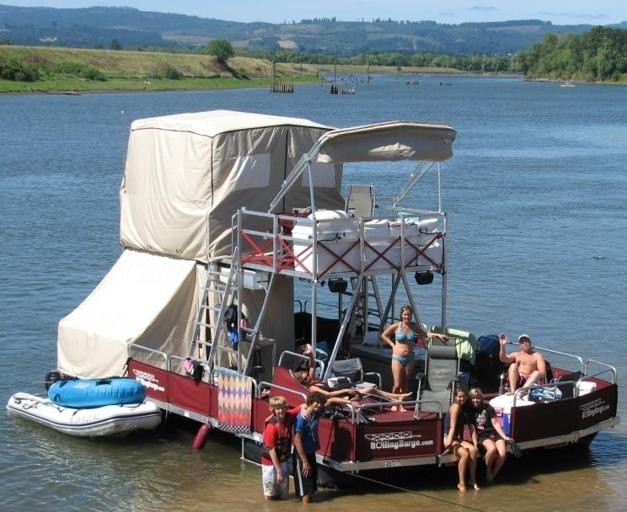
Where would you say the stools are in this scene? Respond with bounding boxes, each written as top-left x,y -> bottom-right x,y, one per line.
240,338 -> 278,377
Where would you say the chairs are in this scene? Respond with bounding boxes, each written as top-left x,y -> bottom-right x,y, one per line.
345,185 -> 376,218
288,368 -> 399,425
414,336 -> 462,418
330,358 -> 382,410
498,361 -> 554,395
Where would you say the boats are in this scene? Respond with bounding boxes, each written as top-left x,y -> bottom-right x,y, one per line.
6,367 -> 162,439
37,110 -> 621,484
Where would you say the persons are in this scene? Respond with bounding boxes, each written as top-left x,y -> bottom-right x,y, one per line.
442,388 -> 480,492
468,387 -> 515,484
497,332 -> 549,399
291,342 -> 413,406
291,388 -> 360,502
379,301 -> 448,412
259,396 -> 310,500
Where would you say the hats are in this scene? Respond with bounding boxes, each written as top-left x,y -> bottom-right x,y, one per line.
518,334 -> 530,342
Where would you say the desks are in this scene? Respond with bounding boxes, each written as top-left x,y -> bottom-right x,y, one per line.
275,212 -> 312,229
